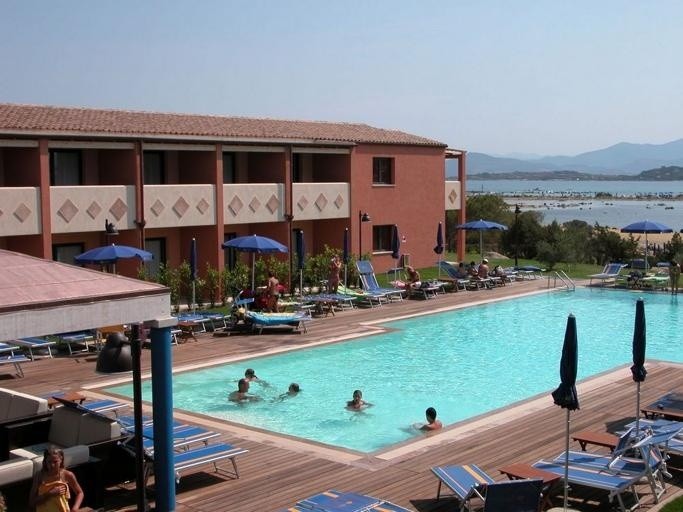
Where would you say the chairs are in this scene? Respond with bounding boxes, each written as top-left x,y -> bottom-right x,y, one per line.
41,390 -> 248,497
585,262 -> 670,295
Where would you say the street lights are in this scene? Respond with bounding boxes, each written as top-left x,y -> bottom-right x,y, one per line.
357,209 -> 372,294
102,219 -> 118,277
512,204 -> 522,272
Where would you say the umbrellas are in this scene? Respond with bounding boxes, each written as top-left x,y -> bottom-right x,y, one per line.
221,234 -> 290,303
624,293 -> 654,455
64,242 -> 154,275
549,310 -> 580,508
456,216 -> 507,271
618,220 -> 676,274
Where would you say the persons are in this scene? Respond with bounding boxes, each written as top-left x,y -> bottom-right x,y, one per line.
278,381 -> 303,399
264,270 -> 280,313
396,405 -> 443,433
345,390 -> 374,411
456,262 -> 468,279
225,378 -> 260,405
475,258 -> 490,280
404,265 -> 422,290
34,443 -> 86,511
237,367 -> 266,387
325,253 -> 343,297
467,260 -> 478,279
